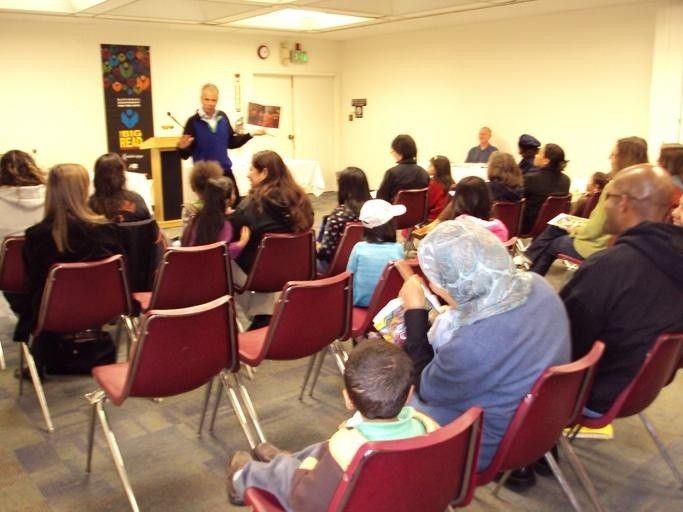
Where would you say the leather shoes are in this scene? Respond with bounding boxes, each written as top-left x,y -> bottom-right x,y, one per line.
496,464 -> 537,490
536,444 -> 561,475
254,443 -> 283,461
226,451 -> 255,506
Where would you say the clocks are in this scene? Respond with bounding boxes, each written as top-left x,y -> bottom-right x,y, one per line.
258,44 -> 271,59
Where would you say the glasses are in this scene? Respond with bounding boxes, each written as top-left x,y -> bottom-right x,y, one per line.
390,149 -> 397,153
606,191 -> 635,201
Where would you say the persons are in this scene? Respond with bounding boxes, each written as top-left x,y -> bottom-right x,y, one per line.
179,176 -> 250,293
14,162 -> 139,351
555,171 -> 608,220
512,137 -> 648,274
464,127 -> 501,163
1,151 -> 55,324
412,151 -> 524,237
398,221 -> 571,468
481,162 -> 682,491
452,178 -> 507,242
376,136 -> 427,202
90,153 -> 168,279
176,83 -> 267,206
229,150 -> 313,329
341,197 -> 407,307
518,145 -> 571,232
311,169 -> 372,267
183,160 -> 222,236
226,340 -> 445,512
512,136 -> 539,176
423,154 -> 455,222
651,144 -> 681,218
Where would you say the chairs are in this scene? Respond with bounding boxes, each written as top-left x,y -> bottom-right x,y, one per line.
236,229 -> 316,294
490,198 -> 525,240
522,194 -> 571,238
559,331 -> 683,512
20,254 -> 134,432
392,185 -> 430,231
132,240 -> 234,310
85,293 -> 254,512
0,235 -> 30,369
244,406 -> 485,512
309,259 -> 430,398
210,270 -> 354,452
476,339 -> 606,512
581,190 -> 602,218
112,217 -> 157,278
318,221 -> 364,277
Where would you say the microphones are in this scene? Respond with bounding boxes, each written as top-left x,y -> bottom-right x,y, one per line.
168,112 -> 185,130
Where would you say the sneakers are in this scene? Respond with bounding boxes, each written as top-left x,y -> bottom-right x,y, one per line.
511,254 -> 533,266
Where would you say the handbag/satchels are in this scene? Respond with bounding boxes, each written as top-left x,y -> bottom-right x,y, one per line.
47,326 -> 116,373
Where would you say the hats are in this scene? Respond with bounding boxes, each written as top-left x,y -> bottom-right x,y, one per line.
519,134 -> 540,148
359,199 -> 407,228
